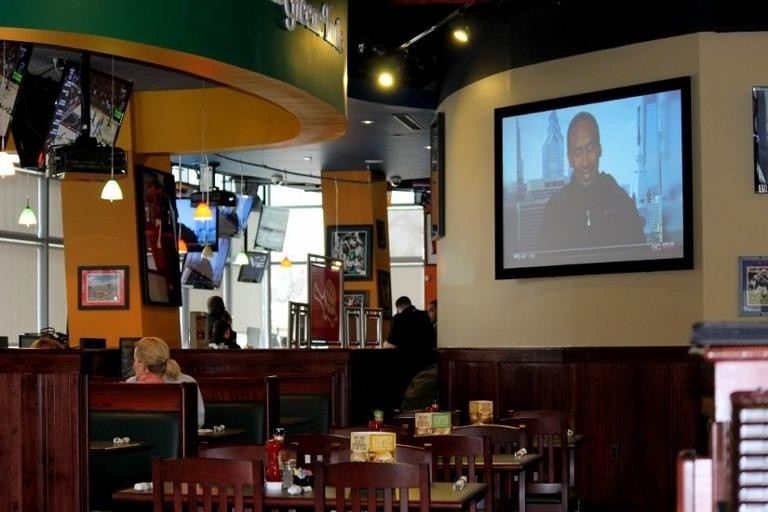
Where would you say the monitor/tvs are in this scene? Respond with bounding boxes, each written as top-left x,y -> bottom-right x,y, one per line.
9,59 -> 136,170
492,75 -> 695,281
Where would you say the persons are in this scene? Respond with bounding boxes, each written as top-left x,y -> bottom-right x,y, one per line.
124,337 -> 205,432
205,296 -> 235,343
426,297 -> 438,331
538,112 -> 648,262
381,296 -> 432,419
208,321 -> 239,351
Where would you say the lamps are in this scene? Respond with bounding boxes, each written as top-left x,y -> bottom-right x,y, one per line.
19,167 -> 36,227
195,81 -> 213,220
202,222 -> 213,259
101,58 -> 123,203
178,156 -> 188,254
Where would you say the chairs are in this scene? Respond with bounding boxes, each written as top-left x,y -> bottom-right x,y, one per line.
508,410 -> 576,512
393,409 -> 461,426
420,434 -> 491,511
295,434 -> 351,463
197,441 -> 267,471
315,459 -> 430,511
329,442 -> 433,482
449,423 -> 526,511
507,417 -> 567,512
151,453 -> 264,511
330,422 -> 409,438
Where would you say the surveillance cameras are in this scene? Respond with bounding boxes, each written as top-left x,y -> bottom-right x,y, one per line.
271,174 -> 288,185
389,174 -> 403,188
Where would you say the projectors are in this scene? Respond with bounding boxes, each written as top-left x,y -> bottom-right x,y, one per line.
48,55 -> 129,181
190,160 -> 238,208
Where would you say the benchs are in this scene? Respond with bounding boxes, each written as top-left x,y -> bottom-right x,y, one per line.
204,401 -> 265,447
89,410 -> 182,510
279,392 -> 332,437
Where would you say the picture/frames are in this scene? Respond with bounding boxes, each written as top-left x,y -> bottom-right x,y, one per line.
326,224 -> 374,281
343,289 -> 370,310
738,256 -> 768,318
430,111 -> 446,240
425,212 -> 437,266
752,85 -> 767,195
135,163 -> 182,306
376,219 -> 386,249
280,257 -> 292,267
378,269 -> 392,321
77,265 -> 130,310
